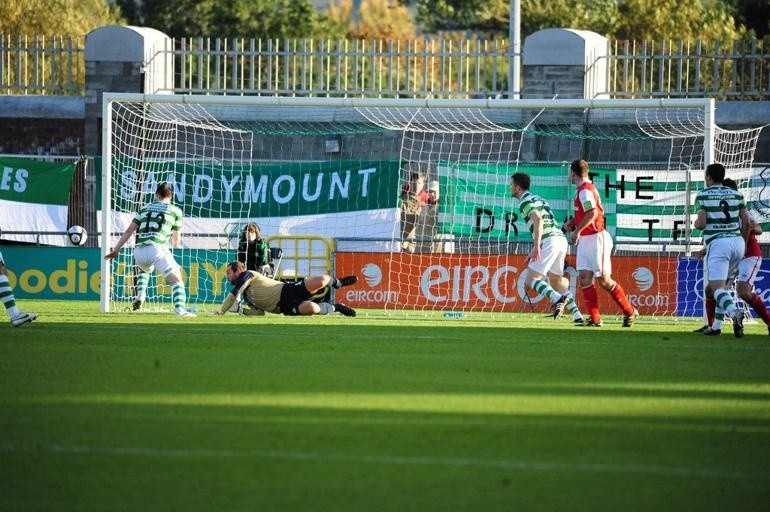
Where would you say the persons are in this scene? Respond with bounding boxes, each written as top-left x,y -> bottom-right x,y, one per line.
212,261 -> 358,318
104,183 -> 197,318
0,228 -> 40,329
510,173 -> 585,327
562,160 -> 640,328
399,171 -> 456,255
236,221 -> 271,315
694,164 -> 751,339
694,179 -> 770,337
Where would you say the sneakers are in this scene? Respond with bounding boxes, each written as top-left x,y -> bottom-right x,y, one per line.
178,311 -> 196,317
576,319 -> 602,327
335,276 -> 357,288
337,304 -> 356,316
11,313 -> 38,326
695,325 -> 721,336
732,310 -> 745,337
133,296 -> 144,310
553,296 -> 569,319
623,309 -> 639,326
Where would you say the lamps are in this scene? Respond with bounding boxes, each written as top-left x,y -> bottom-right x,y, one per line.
324,135 -> 341,154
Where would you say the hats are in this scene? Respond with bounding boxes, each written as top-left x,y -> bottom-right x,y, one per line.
428,181 -> 439,191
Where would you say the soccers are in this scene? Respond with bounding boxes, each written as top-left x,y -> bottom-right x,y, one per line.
67,226 -> 87,247
